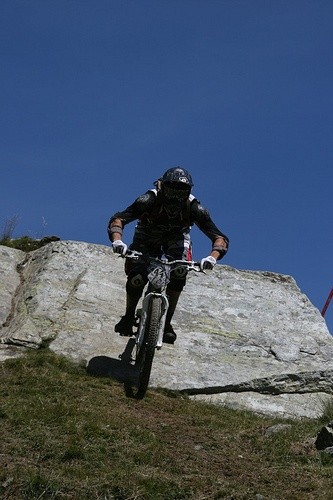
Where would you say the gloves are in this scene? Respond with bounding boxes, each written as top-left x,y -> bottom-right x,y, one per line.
112,240 -> 128,256
200,255 -> 217,272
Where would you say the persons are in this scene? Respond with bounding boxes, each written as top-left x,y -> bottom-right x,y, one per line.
107,168 -> 229,344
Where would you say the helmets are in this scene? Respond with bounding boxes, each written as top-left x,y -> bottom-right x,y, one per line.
163,166 -> 194,189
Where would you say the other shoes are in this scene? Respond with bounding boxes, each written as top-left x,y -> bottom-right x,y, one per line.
163,324 -> 176,345
114,317 -> 134,334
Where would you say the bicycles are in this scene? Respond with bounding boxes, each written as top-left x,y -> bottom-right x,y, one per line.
119,249 -> 206,399
